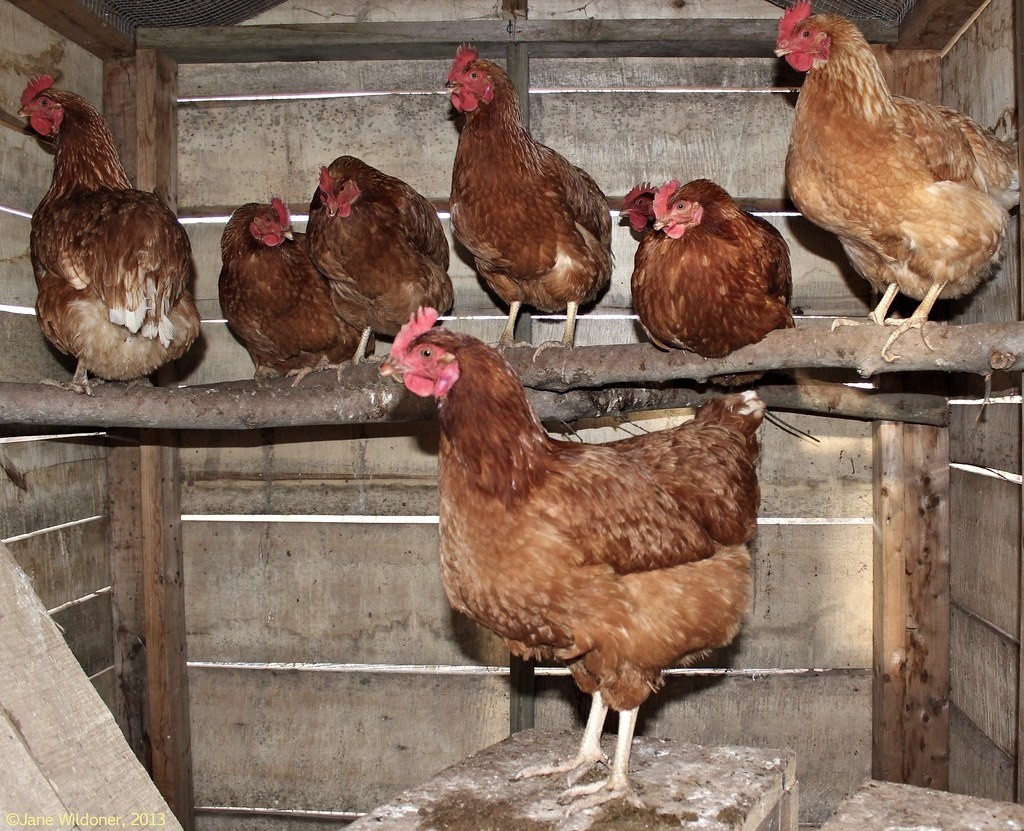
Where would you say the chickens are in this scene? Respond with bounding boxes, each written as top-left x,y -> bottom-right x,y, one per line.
617,176 -> 798,358
217,155 -> 454,390
438,38 -> 616,364
16,74 -> 203,397
378,306 -> 768,819
774,0 -> 1022,365
